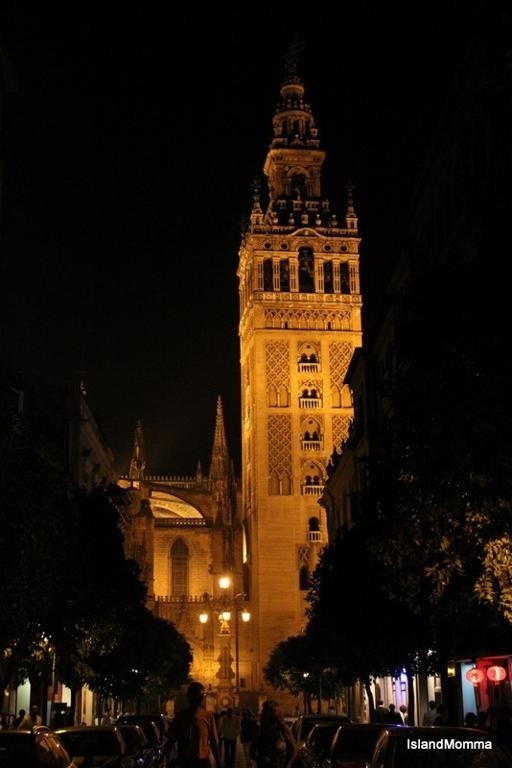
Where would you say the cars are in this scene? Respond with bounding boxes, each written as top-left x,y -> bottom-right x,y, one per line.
282,714 -> 351,767
115,713 -> 177,767
1,725 -> 136,768
320,724 -> 512,768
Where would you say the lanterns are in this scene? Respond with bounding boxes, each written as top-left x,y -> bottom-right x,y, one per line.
465,667 -> 484,688
486,666 -> 506,686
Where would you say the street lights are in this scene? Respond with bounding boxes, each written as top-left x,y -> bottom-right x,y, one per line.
197,568 -> 252,696
